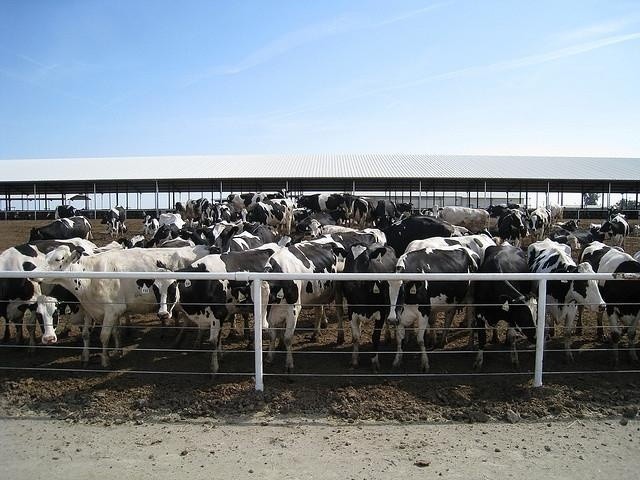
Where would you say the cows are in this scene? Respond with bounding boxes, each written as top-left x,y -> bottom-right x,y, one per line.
0,188 -> 640,381
